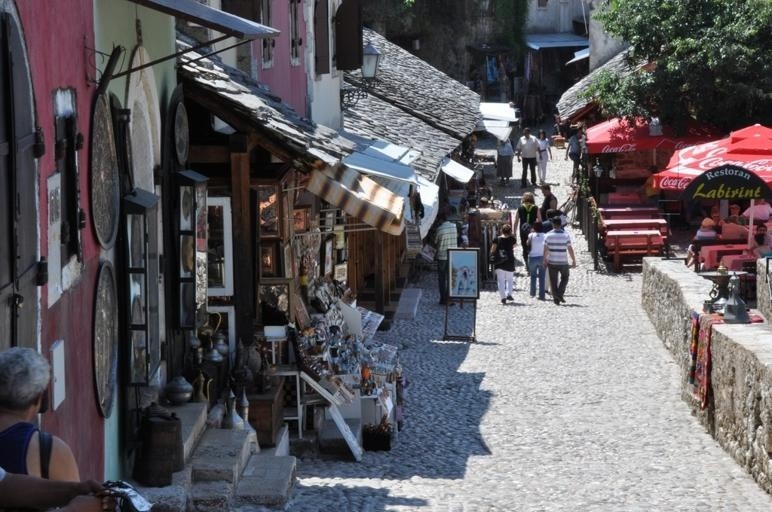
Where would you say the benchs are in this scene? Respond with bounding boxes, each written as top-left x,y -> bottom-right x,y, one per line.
602,215 -> 671,271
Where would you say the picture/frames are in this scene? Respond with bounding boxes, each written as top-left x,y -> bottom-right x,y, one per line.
206,189 -> 237,368
321,221 -> 349,284
447,245 -> 481,303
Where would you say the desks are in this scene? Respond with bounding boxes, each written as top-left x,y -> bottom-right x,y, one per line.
692,239 -> 757,271
597,208 -> 662,220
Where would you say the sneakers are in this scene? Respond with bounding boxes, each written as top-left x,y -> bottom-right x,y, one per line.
555,295 -> 566,304
501,177 -> 509,183
522,181 -> 545,186
502,295 -> 514,303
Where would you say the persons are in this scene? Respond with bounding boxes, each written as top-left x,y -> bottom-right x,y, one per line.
541,208 -> 566,294
538,185 -> 557,222
684,217 -> 717,267
546,216 -> 576,305
516,128 -> 542,188
525,220 -> 547,300
564,127 -> 583,186
431,213 -> 458,303
513,191 -> 544,278
0,346 -> 80,484
489,225 -> 518,305
534,129 -> 552,184
0,465 -> 118,511
495,137 -> 515,184
439,172 -> 512,248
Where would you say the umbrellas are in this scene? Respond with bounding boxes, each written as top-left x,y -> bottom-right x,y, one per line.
582,106 -> 771,258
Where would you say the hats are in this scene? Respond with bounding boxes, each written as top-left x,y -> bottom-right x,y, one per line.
730,204 -> 740,210
551,217 -> 561,224
702,218 -> 716,226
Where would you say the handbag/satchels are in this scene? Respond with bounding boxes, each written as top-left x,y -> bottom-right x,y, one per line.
489,250 -> 497,263
521,223 -> 531,234
92,480 -> 182,511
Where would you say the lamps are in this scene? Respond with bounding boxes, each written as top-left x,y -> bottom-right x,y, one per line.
341,38 -> 384,108
